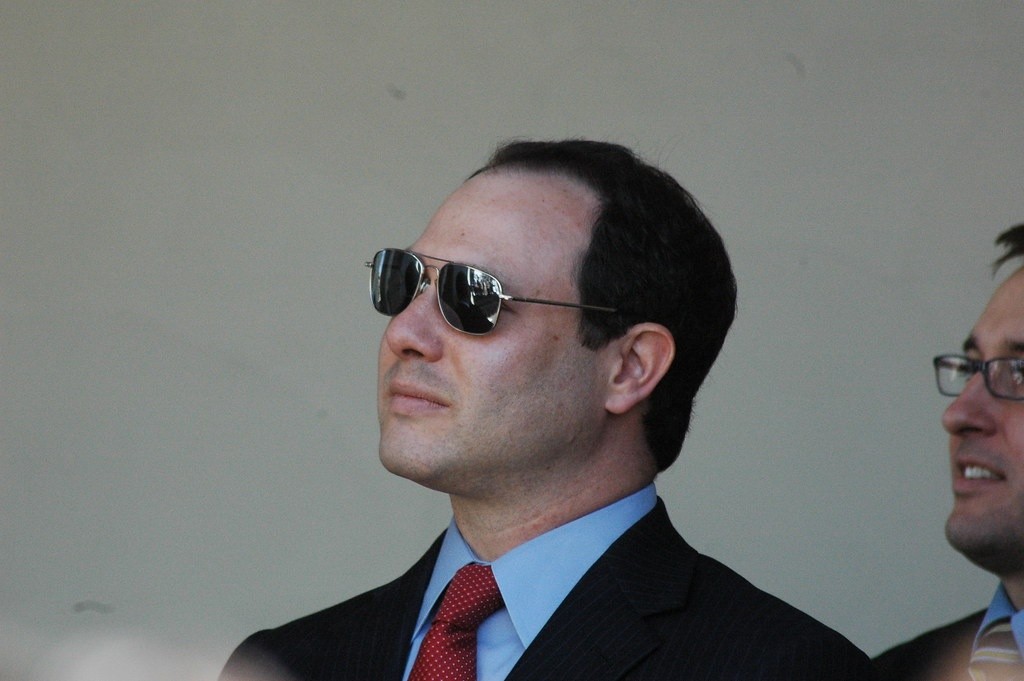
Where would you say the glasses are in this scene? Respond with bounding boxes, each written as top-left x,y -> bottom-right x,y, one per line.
933,354 -> 1024,400
365,247 -> 619,335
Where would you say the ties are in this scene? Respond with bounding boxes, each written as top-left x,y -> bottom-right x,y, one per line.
407,564 -> 505,681
967,618 -> 1021,681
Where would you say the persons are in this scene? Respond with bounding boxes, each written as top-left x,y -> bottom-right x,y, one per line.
398,260 -> 421,314
453,271 -> 494,334
216,140 -> 879,681
867,225 -> 1024,681
